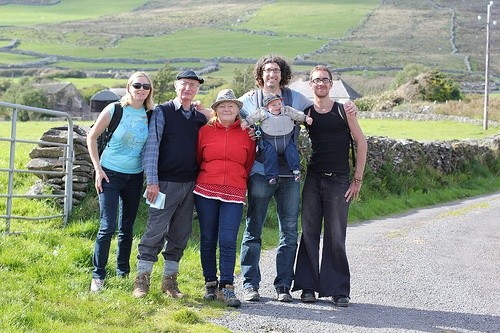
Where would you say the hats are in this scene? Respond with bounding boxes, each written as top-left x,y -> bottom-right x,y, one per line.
176,71 -> 204,85
263,93 -> 283,105
211,89 -> 243,109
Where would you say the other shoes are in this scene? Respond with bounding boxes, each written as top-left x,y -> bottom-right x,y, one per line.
269,179 -> 276,184
334,295 -> 349,307
302,288 -> 315,302
90,278 -> 104,292
295,174 -> 300,181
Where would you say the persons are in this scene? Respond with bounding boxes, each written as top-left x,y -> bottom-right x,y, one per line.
86,70 -> 154,292
194,57 -> 359,301
193,88 -> 255,307
136,70 -> 255,297
291,65 -> 367,307
239,93 -> 313,185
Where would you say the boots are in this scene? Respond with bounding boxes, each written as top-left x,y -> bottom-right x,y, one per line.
134,271 -> 151,296
162,272 -> 184,298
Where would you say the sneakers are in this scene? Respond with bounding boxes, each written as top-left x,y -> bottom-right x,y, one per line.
244,283 -> 260,301
276,287 -> 292,302
220,285 -> 240,307
204,281 -> 218,301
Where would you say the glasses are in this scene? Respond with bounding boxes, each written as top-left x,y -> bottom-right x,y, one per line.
313,78 -> 330,85
262,68 -> 282,73
131,83 -> 151,90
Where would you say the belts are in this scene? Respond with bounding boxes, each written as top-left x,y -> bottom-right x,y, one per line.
324,172 -> 340,176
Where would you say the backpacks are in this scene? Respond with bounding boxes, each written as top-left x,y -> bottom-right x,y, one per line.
97,102 -> 154,156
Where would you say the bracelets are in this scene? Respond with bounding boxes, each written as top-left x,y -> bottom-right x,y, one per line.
352,177 -> 363,183
